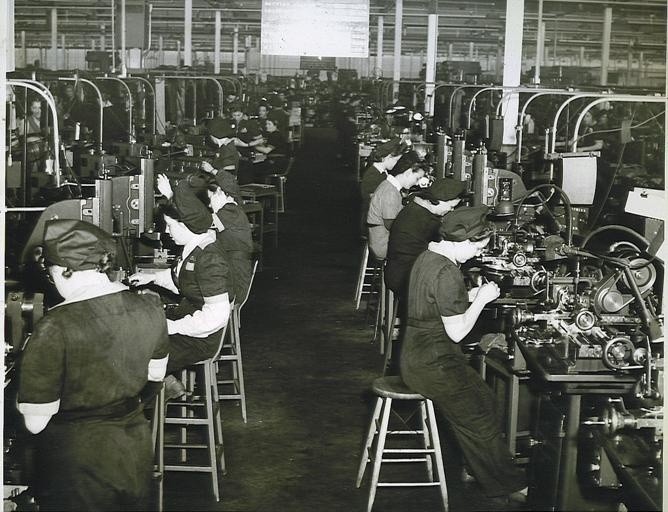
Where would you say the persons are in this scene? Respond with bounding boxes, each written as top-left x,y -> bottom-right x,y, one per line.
398,204 -> 528,504
126,181 -> 234,410
15,216 -> 169,511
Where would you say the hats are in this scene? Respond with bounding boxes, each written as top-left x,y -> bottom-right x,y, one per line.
438,205 -> 490,242
207,116 -> 234,140
170,190 -> 214,235
377,137 -> 401,158
427,176 -> 465,203
391,152 -> 418,177
41,219 -> 118,272
216,169 -> 240,197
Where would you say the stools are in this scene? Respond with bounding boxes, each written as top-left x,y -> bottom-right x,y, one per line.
288,117 -> 303,152
141,303 -> 247,502
232,154 -> 293,250
353,234 -> 408,364
356,376 -> 448,511
